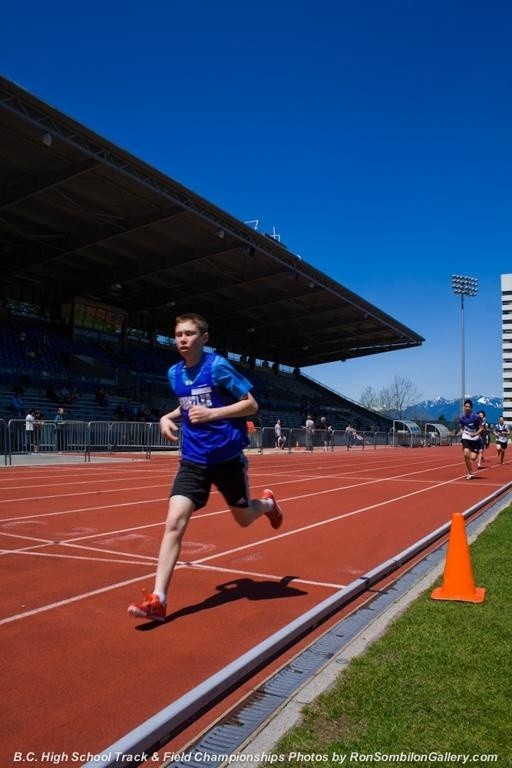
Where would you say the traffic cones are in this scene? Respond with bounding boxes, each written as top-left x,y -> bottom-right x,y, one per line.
430,513 -> 486,604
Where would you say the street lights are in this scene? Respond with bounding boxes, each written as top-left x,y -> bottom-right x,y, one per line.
453,274 -> 478,416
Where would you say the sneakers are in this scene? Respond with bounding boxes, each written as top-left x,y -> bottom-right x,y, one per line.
128,594 -> 166,621
262,489 -> 282,528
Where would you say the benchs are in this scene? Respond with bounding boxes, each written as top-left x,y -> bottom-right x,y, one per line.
259,412 -> 369,431
0,393 -> 158,433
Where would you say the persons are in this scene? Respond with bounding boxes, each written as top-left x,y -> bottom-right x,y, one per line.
127,312 -> 283,623
0,328 -> 357,450
447,399 -> 510,481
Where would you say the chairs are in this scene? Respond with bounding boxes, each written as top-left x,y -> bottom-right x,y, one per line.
0,327 -> 169,377
